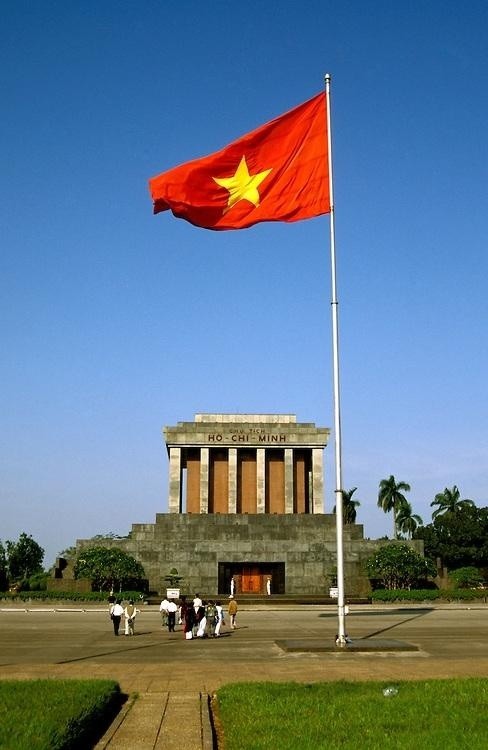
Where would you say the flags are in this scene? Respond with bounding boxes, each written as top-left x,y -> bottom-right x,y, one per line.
149,90 -> 331,231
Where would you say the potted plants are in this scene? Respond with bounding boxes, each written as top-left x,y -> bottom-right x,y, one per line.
164,569 -> 184,599
326,565 -> 345,599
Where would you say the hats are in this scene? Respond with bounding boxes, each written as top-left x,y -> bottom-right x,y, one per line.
228,595 -> 234,599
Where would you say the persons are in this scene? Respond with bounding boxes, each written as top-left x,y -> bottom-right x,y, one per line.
111,601 -> 124,636
108,591 -> 116,614
159,593 -> 238,640
124,601 -> 137,635
